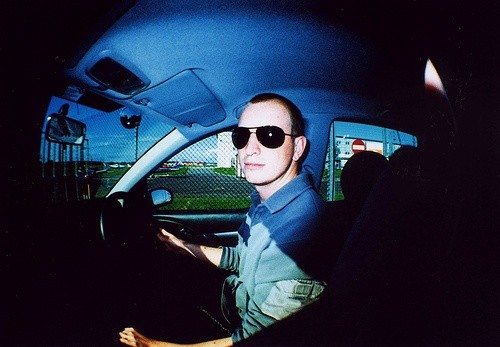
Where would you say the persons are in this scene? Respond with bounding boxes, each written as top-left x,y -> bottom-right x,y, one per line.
118,93 -> 328,347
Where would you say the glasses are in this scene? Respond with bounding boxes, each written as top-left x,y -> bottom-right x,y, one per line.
231,125 -> 296,150
